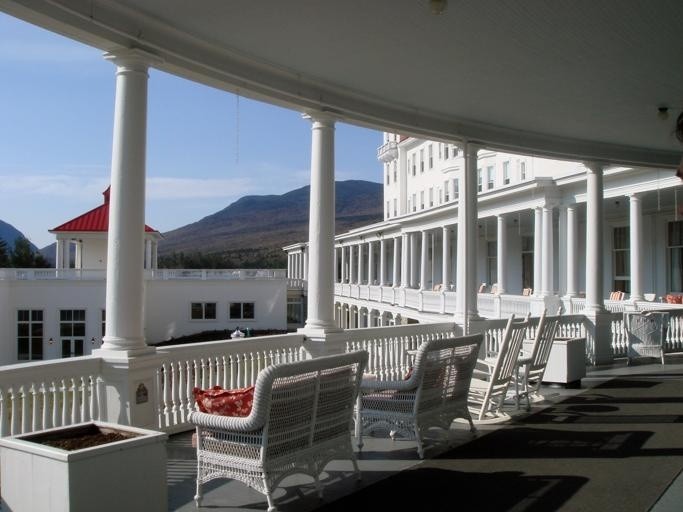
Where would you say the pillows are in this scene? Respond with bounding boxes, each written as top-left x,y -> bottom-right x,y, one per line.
192,387 -> 256,417
667,294 -> 683,305
611,291 -> 622,301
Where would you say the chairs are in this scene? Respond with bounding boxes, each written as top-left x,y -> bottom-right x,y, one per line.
609,292 -> 625,301
659,294 -> 683,304
408,312 -> 533,425
622,311 -> 670,366
644,293 -> 655,301
476,305 -> 563,407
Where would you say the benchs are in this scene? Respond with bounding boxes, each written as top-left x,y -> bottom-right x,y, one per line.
183,349 -> 368,512
349,332 -> 485,458
